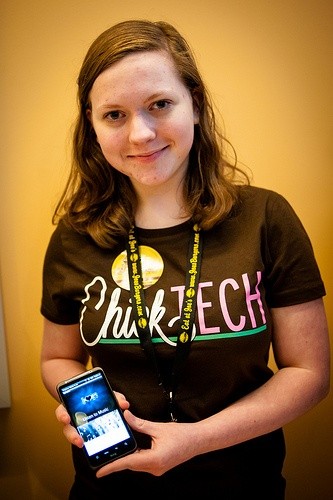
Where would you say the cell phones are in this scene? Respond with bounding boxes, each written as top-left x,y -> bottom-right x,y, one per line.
56,367 -> 137,472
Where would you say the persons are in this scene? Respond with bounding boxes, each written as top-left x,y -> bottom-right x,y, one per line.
40,18 -> 333,500
